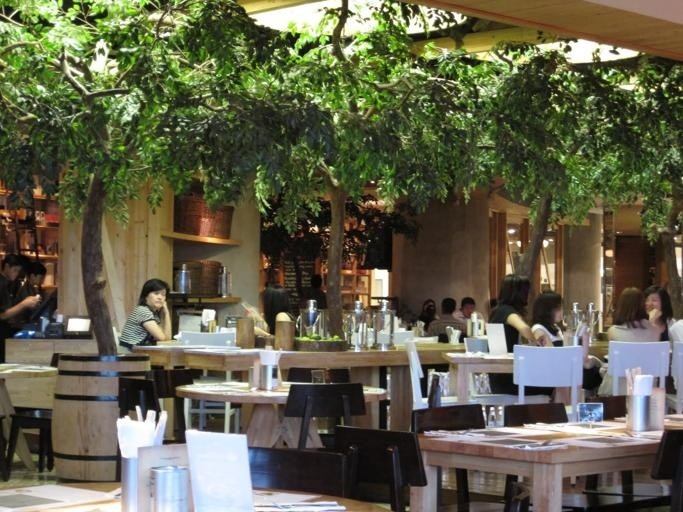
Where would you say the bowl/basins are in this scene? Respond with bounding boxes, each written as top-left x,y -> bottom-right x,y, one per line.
175,196 -> 233,238
173,258 -> 222,296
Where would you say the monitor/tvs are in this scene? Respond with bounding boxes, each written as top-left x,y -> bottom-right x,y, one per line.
50,288 -> 58,313
28,296 -> 52,323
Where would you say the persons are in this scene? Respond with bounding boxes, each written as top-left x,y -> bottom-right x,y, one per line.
264,269 -> 283,289
119,272 -> 173,356
243,283 -> 326,451
414,274 -> 680,398
1,254 -> 46,360
297,273 -> 326,309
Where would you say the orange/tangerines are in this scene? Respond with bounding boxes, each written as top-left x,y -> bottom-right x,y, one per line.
295,333 -> 341,342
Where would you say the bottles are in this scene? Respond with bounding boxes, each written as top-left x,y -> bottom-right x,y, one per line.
217,265 -> 232,298
325,368 -> 333,384
647,387 -> 667,430
349,301 -> 367,349
248,367 -> 255,388
586,302 -> 599,343
568,303 -> 584,333
371,297 -> 394,349
298,300 -> 322,338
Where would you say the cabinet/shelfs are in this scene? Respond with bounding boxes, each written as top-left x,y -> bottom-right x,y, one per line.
316,254 -> 371,307
162,230 -> 242,306
1,209 -> 59,291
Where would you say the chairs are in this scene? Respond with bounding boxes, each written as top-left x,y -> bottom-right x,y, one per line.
0,316 -> 683,511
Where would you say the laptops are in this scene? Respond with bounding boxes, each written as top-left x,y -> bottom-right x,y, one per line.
62,314 -> 93,338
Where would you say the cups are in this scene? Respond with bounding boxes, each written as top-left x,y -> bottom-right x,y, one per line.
208,320 -> 216,332
275,321 -> 296,351
119,456 -> 138,511
173,269 -> 190,293
311,369 -> 325,384
448,331 -> 459,343
151,466 -> 193,512
235,319 -> 255,349
564,336 -> 583,346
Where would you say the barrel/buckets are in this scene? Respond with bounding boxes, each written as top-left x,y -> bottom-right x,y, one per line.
213,204 -> 235,240
173,261 -> 202,294
190,259 -> 224,299
565,309 -> 603,340
173,190 -> 218,237
50,351 -> 150,484
625,393 -> 664,433
286,364 -> 353,445
